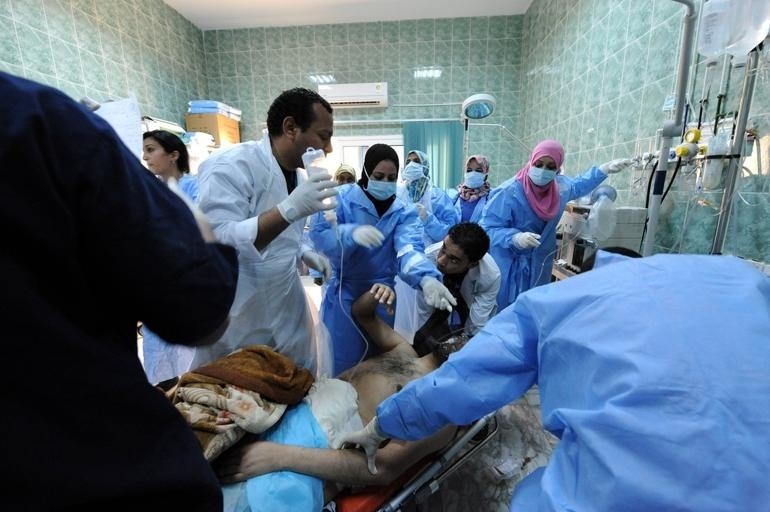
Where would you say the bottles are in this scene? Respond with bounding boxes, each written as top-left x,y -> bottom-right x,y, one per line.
301,148 -> 339,222
483,455 -> 526,484
702,140 -> 727,192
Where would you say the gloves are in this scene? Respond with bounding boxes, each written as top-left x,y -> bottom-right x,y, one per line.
597,157 -> 631,174
277,174 -> 339,225
334,416 -> 390,475
419,274 -> 459,313
512,232 -> 541,250
352,225 -> 385,249
302,249 -> 331,283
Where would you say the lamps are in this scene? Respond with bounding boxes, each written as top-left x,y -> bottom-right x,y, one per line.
460,93 -> 532,175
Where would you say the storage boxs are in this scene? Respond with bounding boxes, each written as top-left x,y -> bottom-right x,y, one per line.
185,113 -> 241,147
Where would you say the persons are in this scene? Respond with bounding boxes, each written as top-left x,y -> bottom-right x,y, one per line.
4,71 -> 770,511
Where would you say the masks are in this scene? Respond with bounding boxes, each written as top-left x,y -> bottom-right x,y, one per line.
464,171 -> 487,189
526,161 -> 558,187
368,178 -> 397,201
404,161 -> 424,183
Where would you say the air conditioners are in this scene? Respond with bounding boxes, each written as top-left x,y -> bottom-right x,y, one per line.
318,81 -> 389,109
